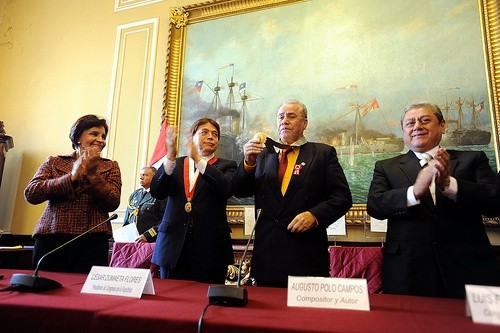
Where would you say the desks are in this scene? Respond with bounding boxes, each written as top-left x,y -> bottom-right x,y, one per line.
0,247 -> 33,271
0,267 -> 500,333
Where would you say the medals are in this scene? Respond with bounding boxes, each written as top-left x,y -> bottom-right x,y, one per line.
185,202 -> 192,213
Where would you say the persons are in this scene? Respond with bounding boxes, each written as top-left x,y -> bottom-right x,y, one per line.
150,118 -> 238,284
367,103 -> 500,299
24,114 -> 122,274
123,167 -> 166,244
231,99 -> 353,288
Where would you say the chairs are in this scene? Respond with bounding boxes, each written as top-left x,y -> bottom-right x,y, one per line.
328,246 -> 383,295
109,242 -> 158,277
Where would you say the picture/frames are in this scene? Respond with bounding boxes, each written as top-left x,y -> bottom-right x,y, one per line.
159,0 -> 500,225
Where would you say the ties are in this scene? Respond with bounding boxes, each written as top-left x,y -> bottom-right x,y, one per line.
278,147 -> 292,191
419,152 -> 431,168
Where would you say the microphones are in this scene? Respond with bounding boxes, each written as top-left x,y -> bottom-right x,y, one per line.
9,214 -> 117,291
208,202 -> 266,306
